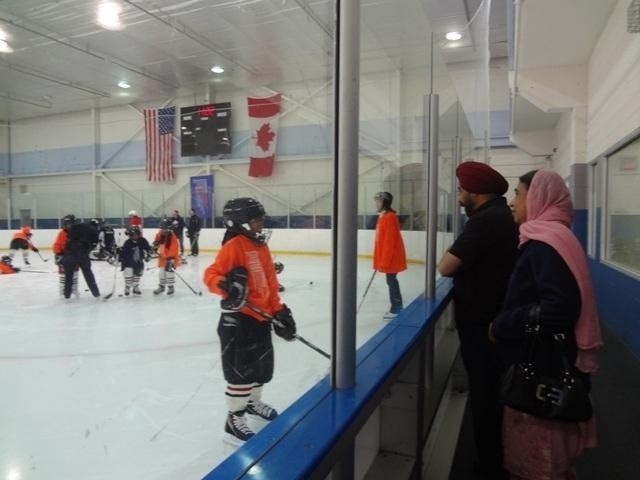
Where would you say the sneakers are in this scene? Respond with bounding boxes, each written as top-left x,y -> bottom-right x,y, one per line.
383,313 -> 398,319
125,285 -> 173,295
224,409 -> 255,441
245,399 -> 277,421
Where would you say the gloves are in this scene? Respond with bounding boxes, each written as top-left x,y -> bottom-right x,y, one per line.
274,304 -> 296,341
221,266 -> 249,310
164,256 -> 176,272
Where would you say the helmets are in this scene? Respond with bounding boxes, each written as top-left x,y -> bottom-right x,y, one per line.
125,224 -> 140,236
223,197 -> 272,246
159,218 -> 174,233
60,215 -> 75,231
374,191 -> 396,213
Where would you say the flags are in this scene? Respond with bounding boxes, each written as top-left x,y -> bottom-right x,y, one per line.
247,94 -> 280,178
180,103 -> 232,154
144,107 -> 175,183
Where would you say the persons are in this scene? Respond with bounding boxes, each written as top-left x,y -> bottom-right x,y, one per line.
188,209 -> 202,256
53,209 -> 151,298
153,207 -> 187,296
0,256 -> 20,275
16,183 -> 33,228
438,161 -> 519,480
487,169 -> 603,480
9,226 -> 37,265
373,192 -> 407,320
204,196 -> 296,446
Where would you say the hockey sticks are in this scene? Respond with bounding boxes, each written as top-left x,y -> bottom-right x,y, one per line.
30,239 -> 50,262
183,237 -> 195,258
20,269 -> 62,274
172,268 -> 202,296
104,254 -> 119,301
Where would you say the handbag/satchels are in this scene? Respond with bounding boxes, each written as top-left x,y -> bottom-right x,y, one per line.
501,362 -> 594,423
